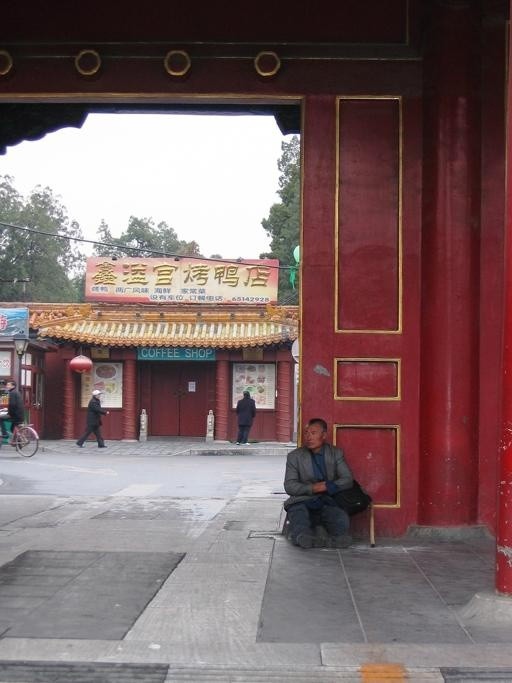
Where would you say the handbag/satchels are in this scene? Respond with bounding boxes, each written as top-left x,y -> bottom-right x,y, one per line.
337,480 -> 373,518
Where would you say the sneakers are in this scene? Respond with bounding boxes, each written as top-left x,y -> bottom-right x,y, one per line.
296,531 -> 352,549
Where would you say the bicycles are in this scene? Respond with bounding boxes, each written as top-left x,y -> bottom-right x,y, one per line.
0,408 -> 39,457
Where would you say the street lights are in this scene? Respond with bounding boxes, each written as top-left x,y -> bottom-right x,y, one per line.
11,330 -> 30,395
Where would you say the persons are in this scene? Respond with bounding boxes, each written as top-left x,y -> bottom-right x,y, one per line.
283,418 -> 354,547
236,389 -> 256,445
75,389 -> 110,447
0,376 -> 25,445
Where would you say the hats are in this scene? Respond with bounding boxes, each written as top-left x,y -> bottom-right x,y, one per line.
92,389 -> 103,397
